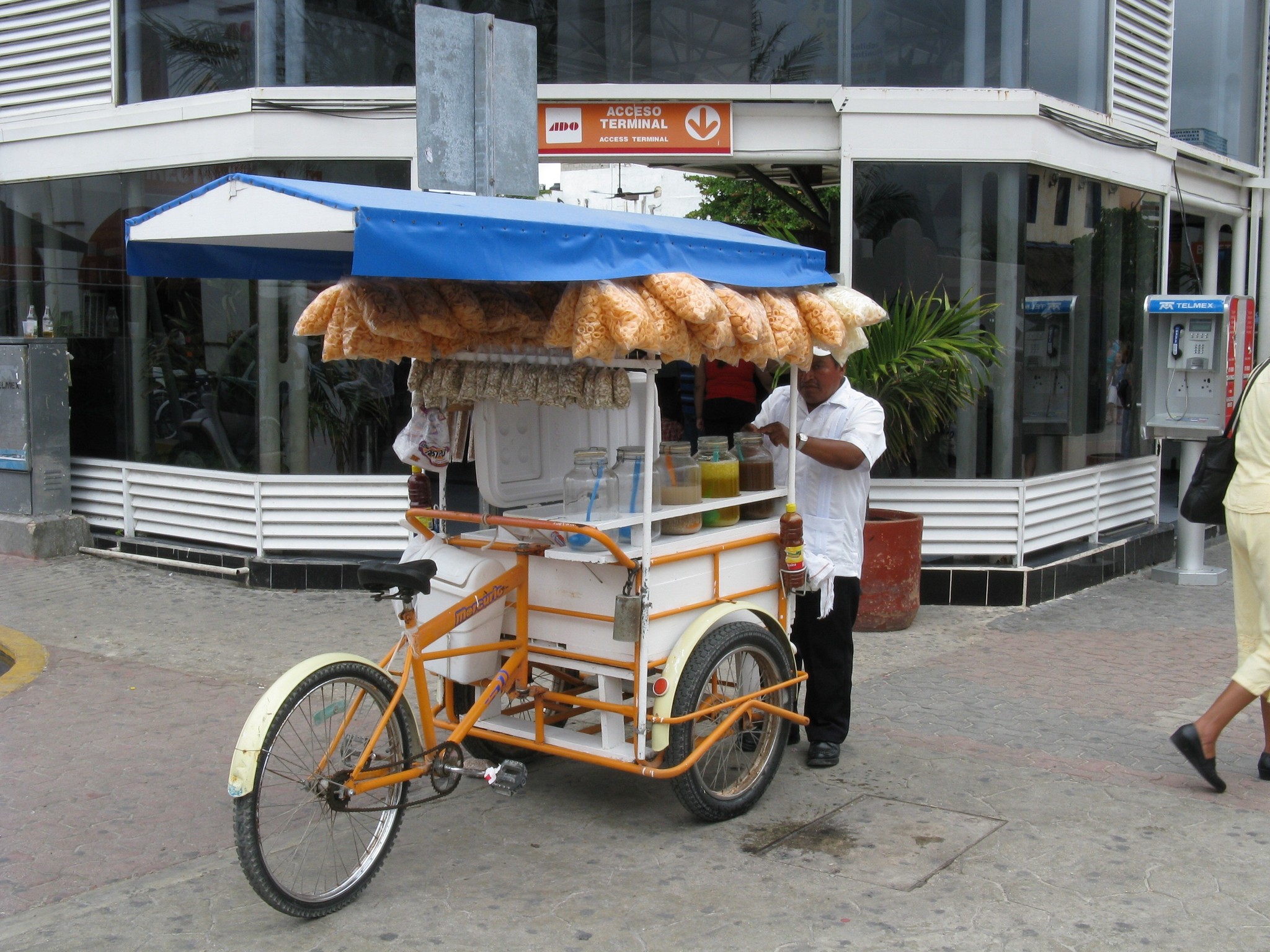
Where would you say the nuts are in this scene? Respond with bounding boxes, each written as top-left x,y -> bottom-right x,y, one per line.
406,358 -> 630,409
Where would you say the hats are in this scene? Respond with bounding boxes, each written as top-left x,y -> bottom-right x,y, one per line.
812,345 -> 832,355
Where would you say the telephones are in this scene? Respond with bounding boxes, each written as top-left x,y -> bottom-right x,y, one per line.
1167,313 -> 1222,372
1024,317 -> 1064,369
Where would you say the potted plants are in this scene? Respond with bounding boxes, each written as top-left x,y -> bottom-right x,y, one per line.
756,217 -> 1008,633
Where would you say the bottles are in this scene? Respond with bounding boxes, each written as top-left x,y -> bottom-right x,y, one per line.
26,305 -> 38,339
691,436 -> 741,527
609,445 -> 662,544
779,503 -> 805,588
728,432 -> 775,520
562,446 -> 619,552
42,305 -> 55,338
407,466 -> 434,536
653,441 -> 702,535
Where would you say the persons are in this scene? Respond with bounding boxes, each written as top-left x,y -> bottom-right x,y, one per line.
739,356 -> 889,764
695,354 -> 778,451
1106,340 -> 1128,425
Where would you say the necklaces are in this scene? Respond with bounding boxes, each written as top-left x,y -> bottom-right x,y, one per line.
1168,358 -> 1270,792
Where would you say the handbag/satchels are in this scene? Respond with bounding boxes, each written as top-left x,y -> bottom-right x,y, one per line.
1180,358 -> 1270,525
392,406 -> 449,473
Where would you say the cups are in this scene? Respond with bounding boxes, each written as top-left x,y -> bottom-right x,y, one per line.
22,321 -> 35,339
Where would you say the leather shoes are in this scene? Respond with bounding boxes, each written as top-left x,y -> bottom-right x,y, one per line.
1258,752 -> 1270,781
1170,723 -> 1227,792
737,728 -> 800,753
805,740 -> 841,766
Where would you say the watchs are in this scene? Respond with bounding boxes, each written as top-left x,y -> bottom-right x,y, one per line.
797,432 -> 809,451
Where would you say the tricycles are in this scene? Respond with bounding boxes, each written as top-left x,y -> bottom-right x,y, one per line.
123,174 -> 810,921
